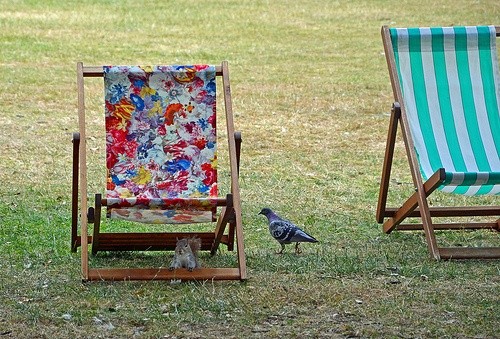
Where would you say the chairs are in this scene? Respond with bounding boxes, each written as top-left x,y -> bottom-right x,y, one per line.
71,61 -> 248,282
375,25 -> 500,262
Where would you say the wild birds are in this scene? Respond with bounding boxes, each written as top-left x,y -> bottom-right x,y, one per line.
258,208 -> 318,254
